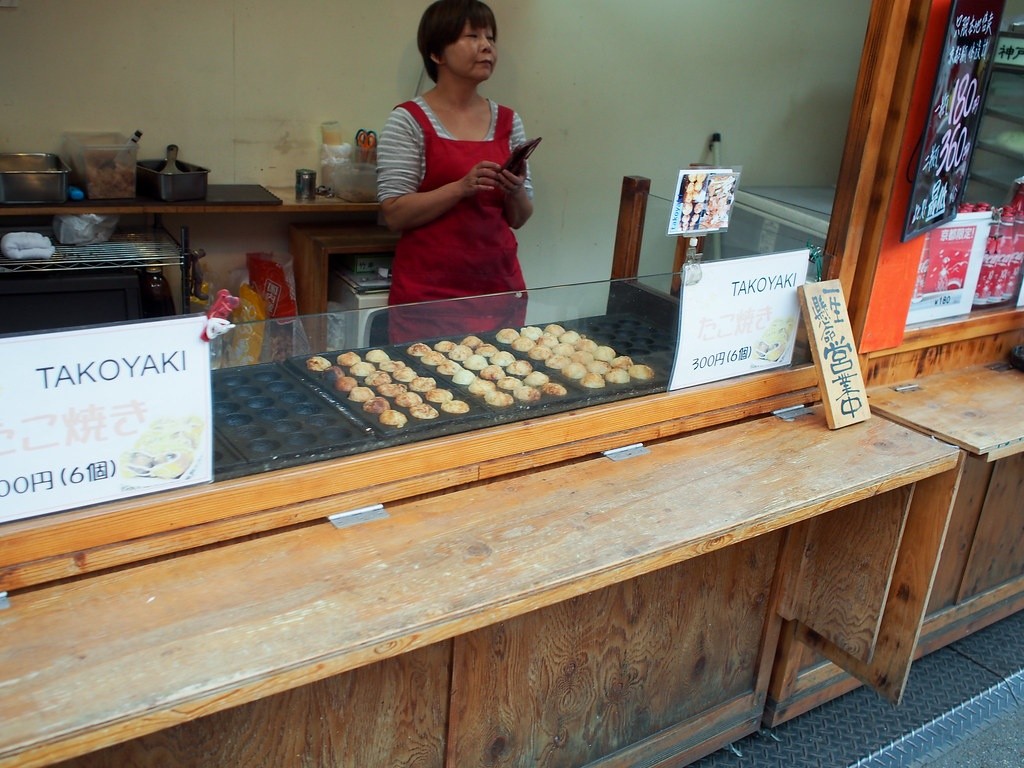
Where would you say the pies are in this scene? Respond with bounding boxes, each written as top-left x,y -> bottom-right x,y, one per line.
308,323 -> 652,431
682,173 -> 705,232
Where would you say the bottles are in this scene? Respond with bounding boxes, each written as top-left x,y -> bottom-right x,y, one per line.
956,202 -> 1024,306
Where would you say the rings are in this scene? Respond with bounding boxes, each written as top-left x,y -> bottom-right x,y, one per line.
476,178 -> 479,185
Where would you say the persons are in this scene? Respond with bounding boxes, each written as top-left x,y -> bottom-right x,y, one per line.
375,0 -> 533,342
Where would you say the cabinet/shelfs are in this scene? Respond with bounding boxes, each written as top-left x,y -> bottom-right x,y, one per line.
963,64 -> 1024,191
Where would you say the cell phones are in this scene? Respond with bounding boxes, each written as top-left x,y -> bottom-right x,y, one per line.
504,137 -> 542,177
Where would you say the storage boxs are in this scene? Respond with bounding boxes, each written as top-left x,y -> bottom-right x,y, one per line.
61,131 -> 140,199
321,161 -> 377,201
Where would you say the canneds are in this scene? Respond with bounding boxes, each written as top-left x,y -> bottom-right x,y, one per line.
296,169 -> 317,199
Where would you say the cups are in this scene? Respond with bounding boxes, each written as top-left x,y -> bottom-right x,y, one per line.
294,168 -> 317,202
